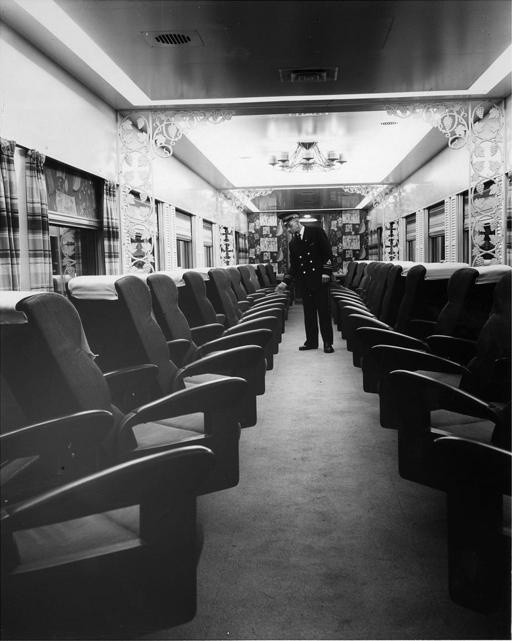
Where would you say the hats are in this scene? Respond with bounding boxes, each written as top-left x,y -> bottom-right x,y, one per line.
277,211 -> 301,226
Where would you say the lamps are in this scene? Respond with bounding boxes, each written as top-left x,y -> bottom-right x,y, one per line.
266,138 -> 346,173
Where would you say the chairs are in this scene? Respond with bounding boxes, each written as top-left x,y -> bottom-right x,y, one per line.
323,257 -> 512,615
0,262 -> 290,640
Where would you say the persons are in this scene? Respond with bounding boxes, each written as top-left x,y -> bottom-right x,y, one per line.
281,214 -> 336,353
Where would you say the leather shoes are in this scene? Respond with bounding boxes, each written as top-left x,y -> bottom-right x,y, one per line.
324,341 -> 335,353
299,341 -> 318,350
